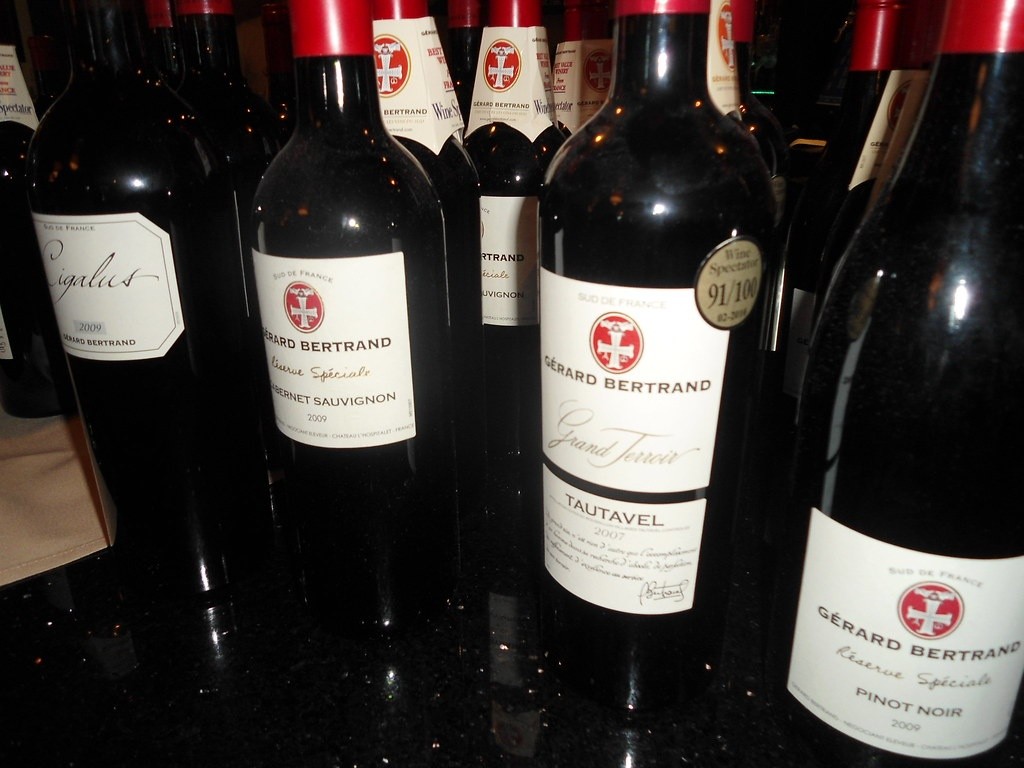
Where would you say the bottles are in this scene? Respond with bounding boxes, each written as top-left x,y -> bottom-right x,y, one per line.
0,1 -> 1024,768
